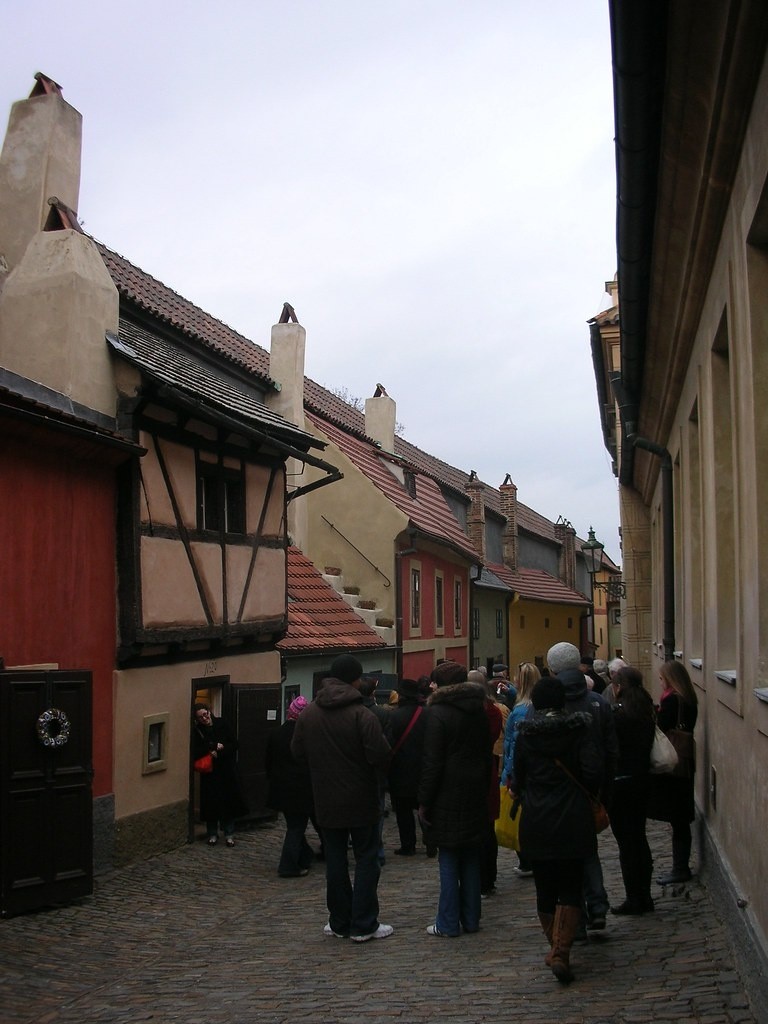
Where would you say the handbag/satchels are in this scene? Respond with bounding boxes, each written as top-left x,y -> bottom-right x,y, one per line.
592,799 -> 608,835
493,785 -> 522,853
195,754 -> 213,773
650,724 -> 679,773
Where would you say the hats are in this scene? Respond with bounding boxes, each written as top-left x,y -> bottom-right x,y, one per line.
331,654 -> 363,685
492,663 -> 509,673
394,679 -> 424,699
547,641 -> 580,676
287,696 -> 309,721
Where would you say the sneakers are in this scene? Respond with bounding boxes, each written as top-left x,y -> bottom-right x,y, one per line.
351,923 -> 394,942
427,923 -> 450,938
324,921 -> 349,938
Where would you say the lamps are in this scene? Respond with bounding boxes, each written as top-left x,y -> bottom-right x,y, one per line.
580,525 -> 627,600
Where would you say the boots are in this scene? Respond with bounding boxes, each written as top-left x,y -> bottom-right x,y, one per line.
550,903 -> 574,979
537,912 -> 556,966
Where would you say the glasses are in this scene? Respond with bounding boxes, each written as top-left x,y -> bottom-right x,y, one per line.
197,710 -> 208,722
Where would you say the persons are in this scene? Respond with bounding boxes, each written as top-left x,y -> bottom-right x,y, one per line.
194,703 -> 238,847
508,677 -> 595,984
267,642 -> 700,942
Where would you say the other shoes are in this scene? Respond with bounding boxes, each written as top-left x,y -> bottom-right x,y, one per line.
427,848 -> 437,858
609,895 -> 654,915
394,845 -> 415,855
280,868 -> 308,878
224,834 -> 234,848
208,830 -> 218,845
655,870 -> 690,886
514,864 -> 534,876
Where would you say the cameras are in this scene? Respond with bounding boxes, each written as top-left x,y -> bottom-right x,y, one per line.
496,686 -> 505,694
